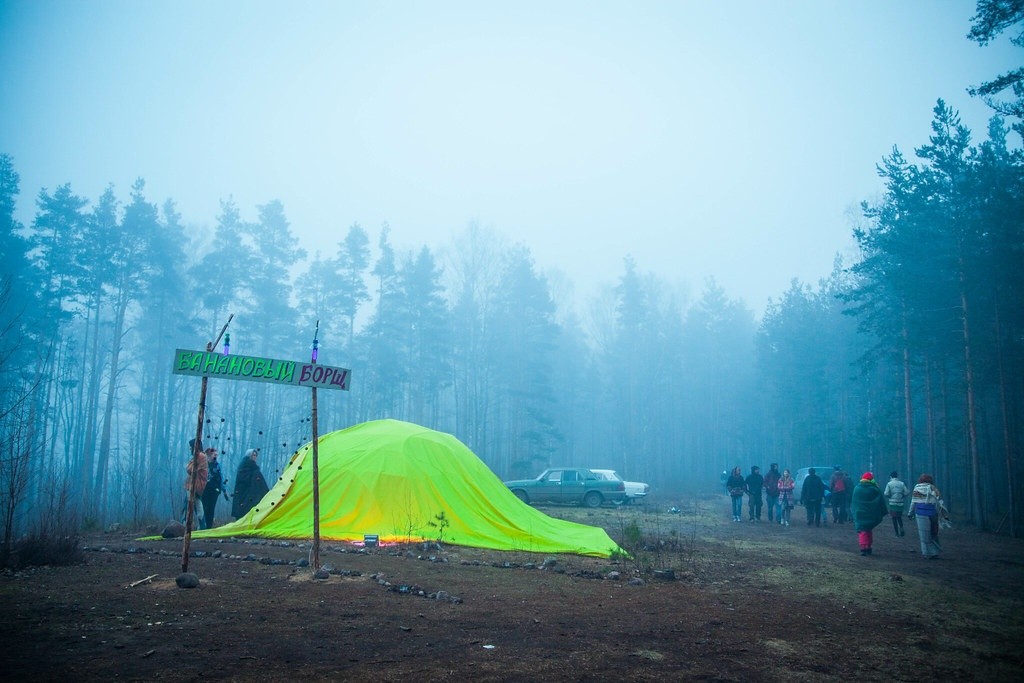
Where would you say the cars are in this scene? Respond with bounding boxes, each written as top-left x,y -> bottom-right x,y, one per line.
792,467 -> 838,505
551,470 -> 650,505
503,468 -> 626,508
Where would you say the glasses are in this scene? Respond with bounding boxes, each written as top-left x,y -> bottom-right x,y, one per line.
253,455 -> 258,457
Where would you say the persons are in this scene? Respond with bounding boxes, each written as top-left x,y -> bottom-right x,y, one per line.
907,474 -> 952,558
884,472 -> 910,536
231,448 -> 269,521
182,437 -> 224,530
720,462 -> 853,526
850,473 -> 887,557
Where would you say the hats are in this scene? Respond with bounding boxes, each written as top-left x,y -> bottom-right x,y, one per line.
862,472 -> 873,480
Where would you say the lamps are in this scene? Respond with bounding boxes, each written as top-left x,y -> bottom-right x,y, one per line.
224,334 -> 230,356
364,534 -> 379,546
313,340 -> 318,360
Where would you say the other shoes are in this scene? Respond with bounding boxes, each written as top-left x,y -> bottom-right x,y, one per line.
808,515 -> 854,526
860,547 -> 872,556
733,515 -> 790,527
895,528 -> 905,537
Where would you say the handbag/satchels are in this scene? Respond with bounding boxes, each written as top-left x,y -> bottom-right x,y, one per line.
206,473 -> 222,494
915,503 -> 936,516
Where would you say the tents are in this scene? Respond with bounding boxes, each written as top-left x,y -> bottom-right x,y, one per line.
135,419 -> 632,560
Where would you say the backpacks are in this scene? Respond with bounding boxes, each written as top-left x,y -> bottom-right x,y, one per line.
835,480 -> 845,492
